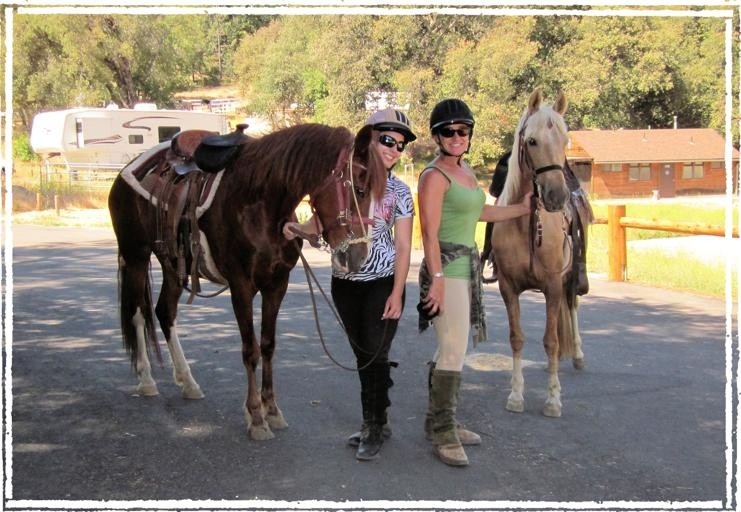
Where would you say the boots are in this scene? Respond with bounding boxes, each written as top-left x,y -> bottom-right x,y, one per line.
424,360 -> 481,465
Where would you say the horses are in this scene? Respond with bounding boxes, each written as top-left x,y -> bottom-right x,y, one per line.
108,123 -> 387,443
492,87 -> 596,417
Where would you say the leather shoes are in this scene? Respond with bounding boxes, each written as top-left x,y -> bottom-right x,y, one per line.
347,423 -> 393,460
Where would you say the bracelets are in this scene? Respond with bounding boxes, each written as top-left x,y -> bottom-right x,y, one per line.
430,271 -> 445,279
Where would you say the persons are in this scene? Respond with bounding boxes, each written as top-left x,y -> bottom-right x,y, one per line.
281,107 -> 415,460
418,98 -> 542,466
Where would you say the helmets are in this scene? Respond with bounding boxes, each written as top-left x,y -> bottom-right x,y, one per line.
430,99 -> 475,136
364,108 -> 417,146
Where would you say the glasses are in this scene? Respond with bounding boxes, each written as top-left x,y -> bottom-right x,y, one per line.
370,135 -> 406,152
436,127 -> 470,138
